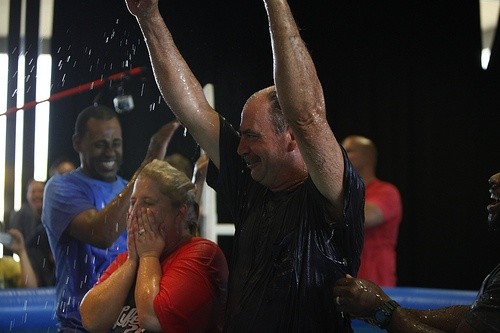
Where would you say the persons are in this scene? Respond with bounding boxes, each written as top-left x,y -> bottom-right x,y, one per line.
340,135 -> 401,288
79,158 -> 229,333
335,172 -> 500,333
40,105 -> 210,333
125,0 -> 365,333
0,158 -> 75,290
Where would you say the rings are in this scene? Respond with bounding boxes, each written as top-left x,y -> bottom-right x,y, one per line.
335,296 -> 340,304
137,227 -> 146,234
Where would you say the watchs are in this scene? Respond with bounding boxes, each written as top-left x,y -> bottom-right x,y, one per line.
371,300 -> 401,329
